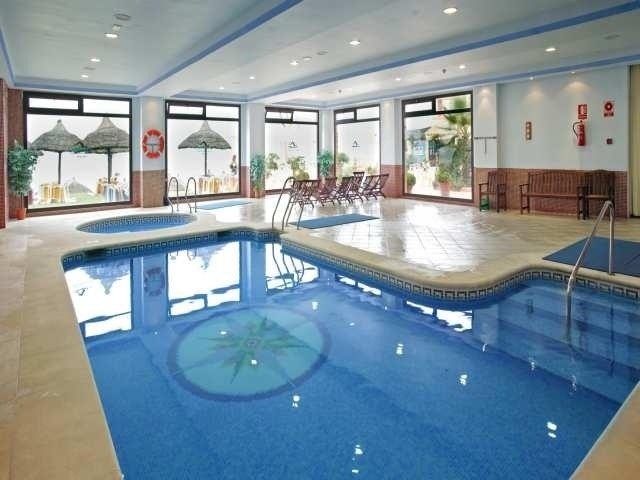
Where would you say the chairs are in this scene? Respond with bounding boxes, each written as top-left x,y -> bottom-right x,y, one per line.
291,173 -> 389,208
582,169 -> 614,220
478,168 -> 507,212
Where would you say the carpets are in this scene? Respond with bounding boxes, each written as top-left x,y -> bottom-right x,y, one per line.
543,235 -> 640,278
289,213 -> 379,228
189,199 -> 253,210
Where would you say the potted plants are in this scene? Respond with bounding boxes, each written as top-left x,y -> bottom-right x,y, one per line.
250,154 -> 280,198
7,142 -> 44,219
438,171 -> 451,197
407,172 -> 416,193
316,150 -> 333,184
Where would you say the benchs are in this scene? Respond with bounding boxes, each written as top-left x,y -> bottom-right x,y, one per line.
518,172 -> 585,220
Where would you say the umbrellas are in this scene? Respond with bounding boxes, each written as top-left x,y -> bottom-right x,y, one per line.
178,120 -> 231,176
83,117 -> 129,184
29,120 -> 83,184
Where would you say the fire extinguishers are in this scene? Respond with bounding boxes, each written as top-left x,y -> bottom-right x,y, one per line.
573,120 -> 585,146
